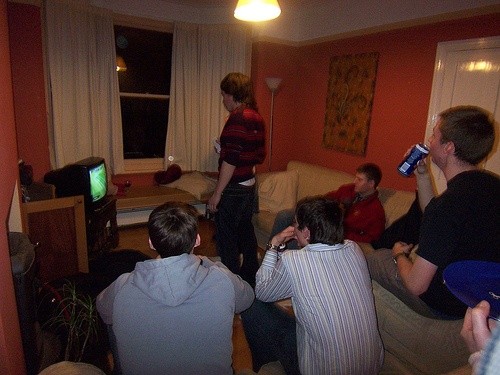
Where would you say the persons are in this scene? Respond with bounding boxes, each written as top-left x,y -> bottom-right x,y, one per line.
97,201 -> 255,375
207,72 -> 266,290
269,163 -> 387,258
362,105 -> 500,375
243,195 -> 386,375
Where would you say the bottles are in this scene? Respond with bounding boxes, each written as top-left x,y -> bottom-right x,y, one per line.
277,242 -> 286,260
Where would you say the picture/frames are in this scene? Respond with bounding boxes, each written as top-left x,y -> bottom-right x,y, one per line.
323,52 -> 379,157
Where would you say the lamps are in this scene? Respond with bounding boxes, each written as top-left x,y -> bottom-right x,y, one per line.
264,78 -> 281,172
234,0 -> 281,22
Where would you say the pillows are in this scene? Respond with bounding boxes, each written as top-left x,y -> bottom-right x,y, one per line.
259,171 -> 299,212
167,174 -> 217,200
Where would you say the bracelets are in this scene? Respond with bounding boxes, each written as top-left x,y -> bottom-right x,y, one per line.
216,187 -> 222,194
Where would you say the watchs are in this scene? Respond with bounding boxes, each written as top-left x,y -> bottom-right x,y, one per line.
267,242 -> 277,250
392,252 -> 410,264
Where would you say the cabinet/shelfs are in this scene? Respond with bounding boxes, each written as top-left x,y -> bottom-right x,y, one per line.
21,180 -> 89,284
92,195 -> 119,256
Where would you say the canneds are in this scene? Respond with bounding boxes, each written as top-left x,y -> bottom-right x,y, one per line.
397,143 -> 430,177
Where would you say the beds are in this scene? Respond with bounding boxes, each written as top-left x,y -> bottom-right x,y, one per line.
117,186 -> 214,220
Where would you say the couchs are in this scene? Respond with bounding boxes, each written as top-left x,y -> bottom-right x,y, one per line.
373,280 -> 500,375
252,161 -> 418,251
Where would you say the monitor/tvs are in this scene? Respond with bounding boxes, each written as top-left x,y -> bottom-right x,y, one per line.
43,157 -> 108,205
